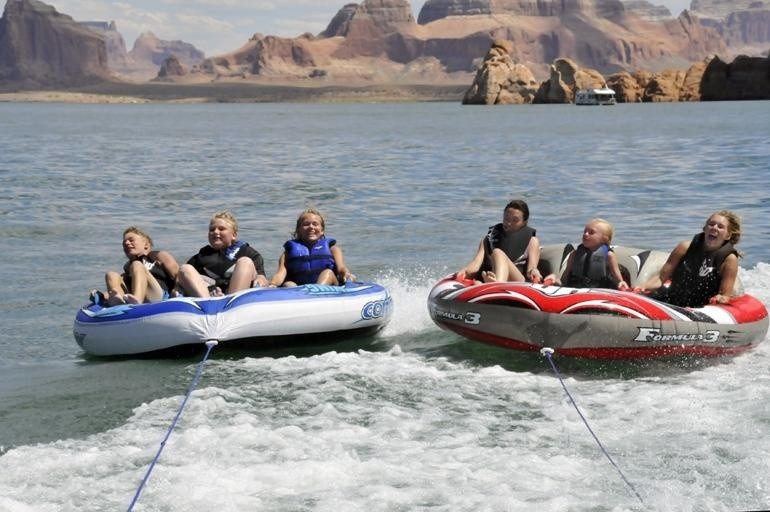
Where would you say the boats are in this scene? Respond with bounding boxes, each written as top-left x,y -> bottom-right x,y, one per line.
74,281 -> 393,360
427,243 -> 769,360
575,88 -> 616,105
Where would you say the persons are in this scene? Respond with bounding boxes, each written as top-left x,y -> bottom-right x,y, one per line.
453,200 -> 543,285
269,208 -> 351,288
90,228 -> 179,306
171,212 -> 269,297
633,210 -> 744,308
543,219 -> 629,291
